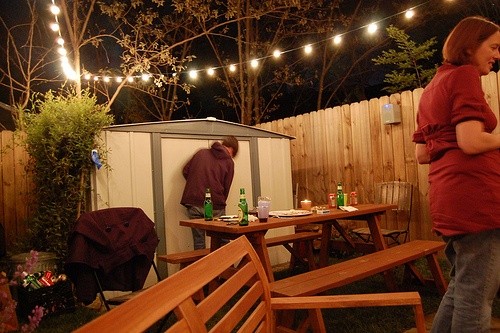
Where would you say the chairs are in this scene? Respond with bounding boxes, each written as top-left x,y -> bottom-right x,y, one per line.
76,206 -> 163,312
352,181 -> 413,280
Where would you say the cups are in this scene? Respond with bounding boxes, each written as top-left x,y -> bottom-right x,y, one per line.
257,206 -> 268,223
258,192 -> 272,211
343,193 -> 348,207
301,201 -> 312,212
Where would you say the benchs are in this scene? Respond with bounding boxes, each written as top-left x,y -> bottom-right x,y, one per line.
70,235 -> 428,333
264,238 -> 448,329
158,230 -> 321,308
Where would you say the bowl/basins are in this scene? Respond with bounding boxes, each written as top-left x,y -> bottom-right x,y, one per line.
248,209 -> 258,218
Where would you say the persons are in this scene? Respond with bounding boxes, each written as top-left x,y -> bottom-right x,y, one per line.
412,15 -> 500,333
180,136 -> 238,250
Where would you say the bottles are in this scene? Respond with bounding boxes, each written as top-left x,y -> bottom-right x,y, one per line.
238,188 -> 248,226
328,193 -> 336,208
16,268 -> 46,290
337,180 -> 343,209
204,188 -> 213,221
350,192 -> 358,207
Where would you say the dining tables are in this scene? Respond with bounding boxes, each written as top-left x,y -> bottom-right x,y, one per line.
179,204 -> 404,290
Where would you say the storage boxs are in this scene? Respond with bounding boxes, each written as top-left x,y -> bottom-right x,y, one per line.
8,271 -> 74,327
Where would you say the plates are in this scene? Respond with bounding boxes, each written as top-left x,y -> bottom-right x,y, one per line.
220,215 -> 258,221
270,210 -> 313,217
